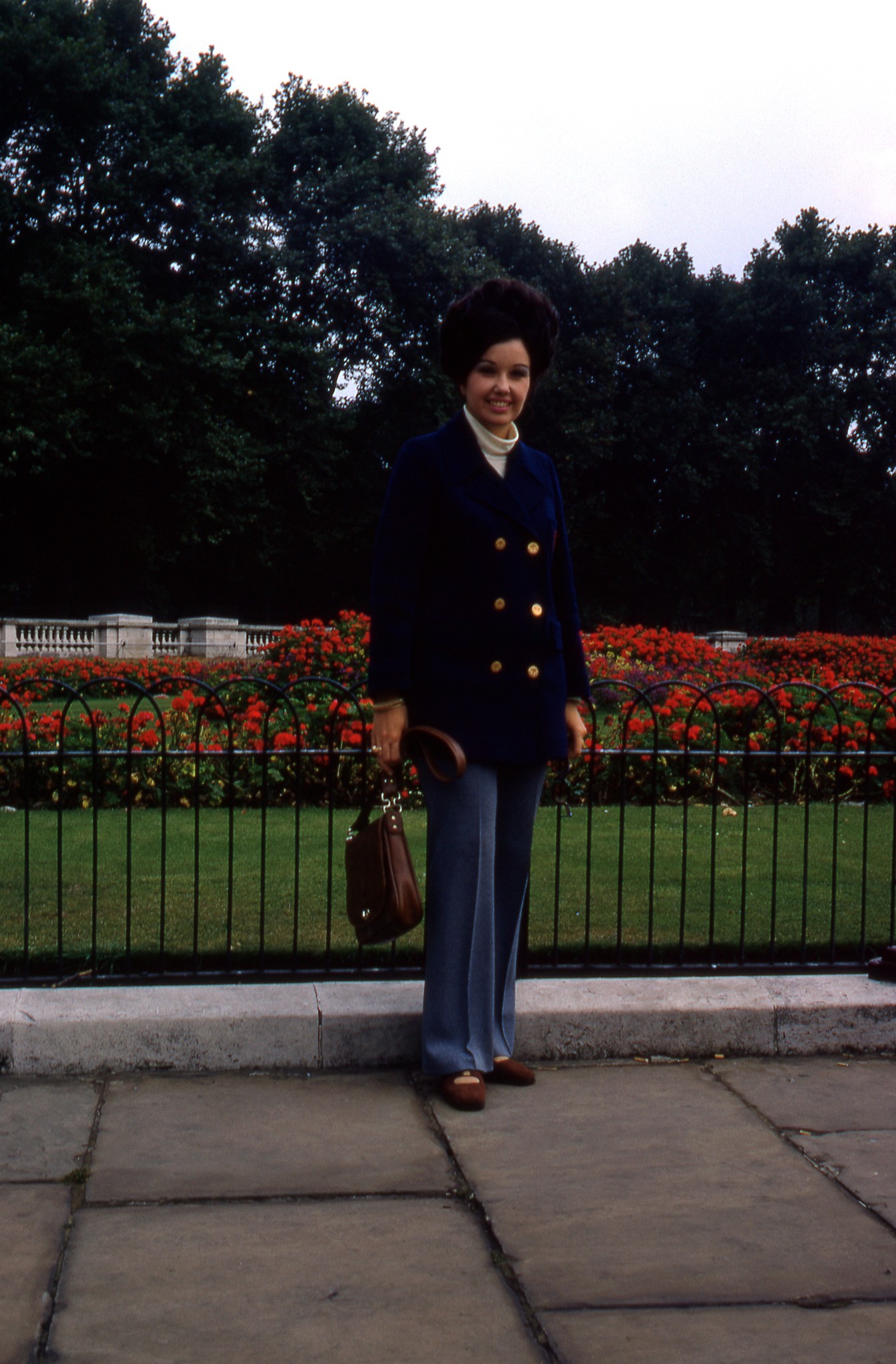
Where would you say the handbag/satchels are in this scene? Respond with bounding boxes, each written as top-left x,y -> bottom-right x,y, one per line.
342,802 -> 425,946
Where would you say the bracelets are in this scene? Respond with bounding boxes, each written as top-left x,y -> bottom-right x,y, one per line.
369,698 -> 405,712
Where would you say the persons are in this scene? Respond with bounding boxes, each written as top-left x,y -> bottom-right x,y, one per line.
367,276 -> 591,1110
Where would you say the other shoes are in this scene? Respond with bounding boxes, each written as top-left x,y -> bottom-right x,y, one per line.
488,1058 -> 536,1084
443,1063 -> 486,1111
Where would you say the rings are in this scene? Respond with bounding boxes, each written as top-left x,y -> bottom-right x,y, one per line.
373,745 -> 382,753
